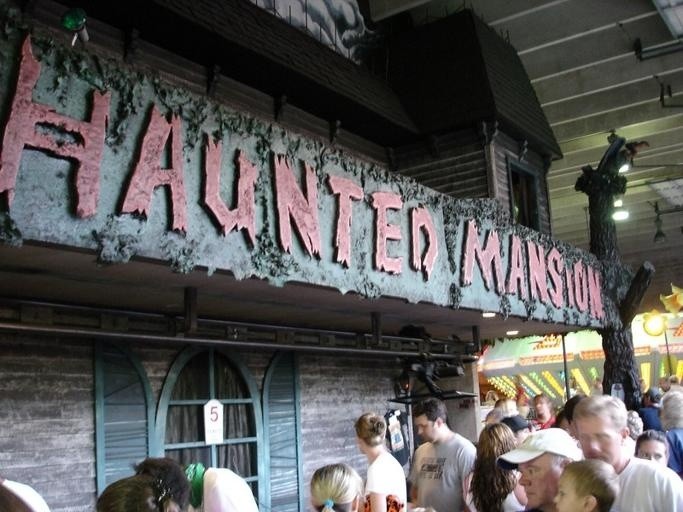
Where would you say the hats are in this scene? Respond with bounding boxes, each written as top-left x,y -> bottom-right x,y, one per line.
495,427 -> 586,470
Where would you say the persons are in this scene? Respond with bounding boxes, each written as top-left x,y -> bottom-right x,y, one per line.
465,375 -> 683,512
96,458 -> 258,512
355,398 -> 478,512
310,462 -> 363,512
